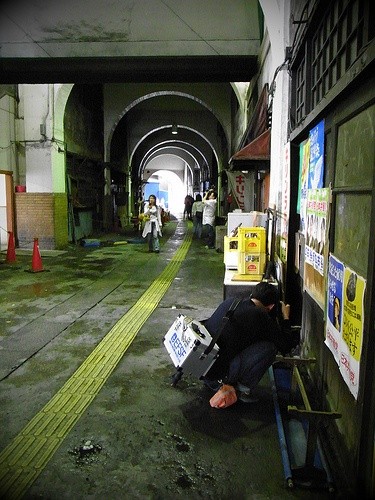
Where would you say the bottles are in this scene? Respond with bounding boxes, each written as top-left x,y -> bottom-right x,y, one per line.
229,223 -> 242,237
229,232 -> 258,263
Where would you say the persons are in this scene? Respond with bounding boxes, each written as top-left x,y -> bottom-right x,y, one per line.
138,195 -> 162,253
162,282 -> 292,403
181,195 -> 195,221
191,194 -> 204,240
202,189 -> 218,249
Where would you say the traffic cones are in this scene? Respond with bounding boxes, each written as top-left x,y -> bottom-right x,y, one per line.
2,231 -> 20,263
24,237 -> 50,273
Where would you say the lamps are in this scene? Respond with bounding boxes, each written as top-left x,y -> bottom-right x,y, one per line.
171,111 -> 178,135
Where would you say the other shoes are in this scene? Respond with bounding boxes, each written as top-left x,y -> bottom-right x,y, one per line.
237,390 -> 261,403
207,380 -> 221,392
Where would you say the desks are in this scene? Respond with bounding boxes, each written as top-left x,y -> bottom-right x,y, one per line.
223,271 -> 279,301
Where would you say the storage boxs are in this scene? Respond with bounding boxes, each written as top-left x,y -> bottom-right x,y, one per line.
238,252 -> 266,275
224,235 -> 238,270
162,313 -> 224,381
238,227 -> 266,252
